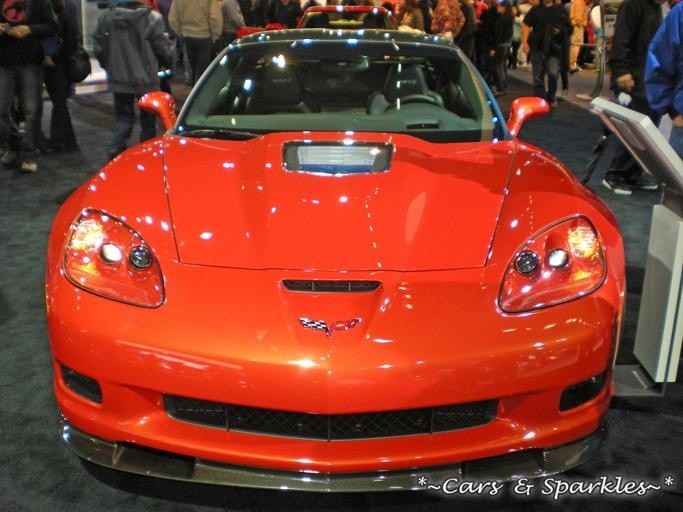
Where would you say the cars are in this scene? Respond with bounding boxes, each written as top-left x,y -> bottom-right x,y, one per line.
266,3 -> 402,31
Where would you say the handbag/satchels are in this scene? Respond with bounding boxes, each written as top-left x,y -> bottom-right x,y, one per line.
528,32 -> 537,49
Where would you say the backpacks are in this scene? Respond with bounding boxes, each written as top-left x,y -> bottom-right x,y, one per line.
56,45 -> 92,83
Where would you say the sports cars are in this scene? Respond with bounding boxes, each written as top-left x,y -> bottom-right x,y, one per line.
35,24 -> 637,506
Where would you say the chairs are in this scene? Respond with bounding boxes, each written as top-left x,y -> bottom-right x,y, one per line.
244,65 -> 313,113
369,66 -> 441,110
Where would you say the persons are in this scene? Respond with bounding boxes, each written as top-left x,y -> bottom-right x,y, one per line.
643,1 -> 683,159
3,0 -> 602,173
597,0 -> 675,197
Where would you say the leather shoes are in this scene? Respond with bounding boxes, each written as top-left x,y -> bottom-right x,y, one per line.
551,101 -> 558,107
570,68 -> 579,74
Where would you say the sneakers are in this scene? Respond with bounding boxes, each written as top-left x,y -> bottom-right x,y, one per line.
0,149 -> 17,164
490,85 -> 496,92
108,143 -> 127,155
601,173 -> 633,195
63,143 -> 84,160
561,89 -> 568,97
494,87 -> 508,97
633,170 -> 659,191
21,140 -> 65,172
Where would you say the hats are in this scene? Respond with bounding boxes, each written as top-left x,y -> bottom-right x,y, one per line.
496,0 -> 506,5
